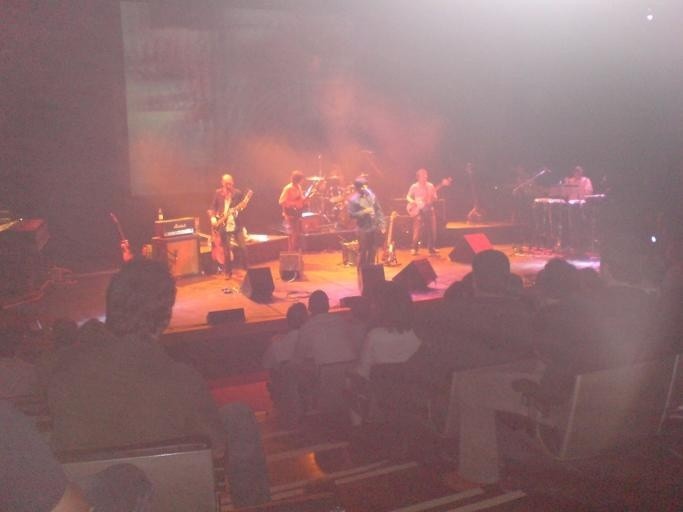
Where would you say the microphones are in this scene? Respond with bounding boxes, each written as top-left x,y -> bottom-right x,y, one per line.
173,249 -> 178,261
541,166 -> 552,173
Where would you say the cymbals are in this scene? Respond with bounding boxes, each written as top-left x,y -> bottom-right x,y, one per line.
306,177 -> 324,181
560,185 -> 579,187
326,176 -> 340,179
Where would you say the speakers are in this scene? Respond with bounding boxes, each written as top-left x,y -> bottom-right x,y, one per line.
152,233 -> 201,280
392,258 -> 438,291
240,268 -> 274,300
448,232 -> 493,261
279,253 -> 303,281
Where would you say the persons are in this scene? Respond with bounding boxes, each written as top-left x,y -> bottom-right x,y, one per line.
2,253 -> 270,509
277,168 -> 318,252
560,165 -> 593,200
346,174 -> 389,270
267,237 -> 683,492
404,168 -> 442,257
208,172 -> 252,282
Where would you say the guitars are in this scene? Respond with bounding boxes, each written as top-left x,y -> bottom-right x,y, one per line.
382,210 -> 399,262
108,208 -> 134,263
286,182 -> 316,220
207,208 -> 233,265
407,176 -> 453,218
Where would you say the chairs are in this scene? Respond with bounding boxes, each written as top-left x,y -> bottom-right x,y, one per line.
57,438 -> 222,511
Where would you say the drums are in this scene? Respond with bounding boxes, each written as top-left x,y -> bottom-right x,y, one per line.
586,194 -> 605,257
300,212 -> 320,232
532,199 -> 548,251
336,204 -> 358,229
548,199 -> 568,252
568,200 -> 586,257
328,195 -> 344,210
